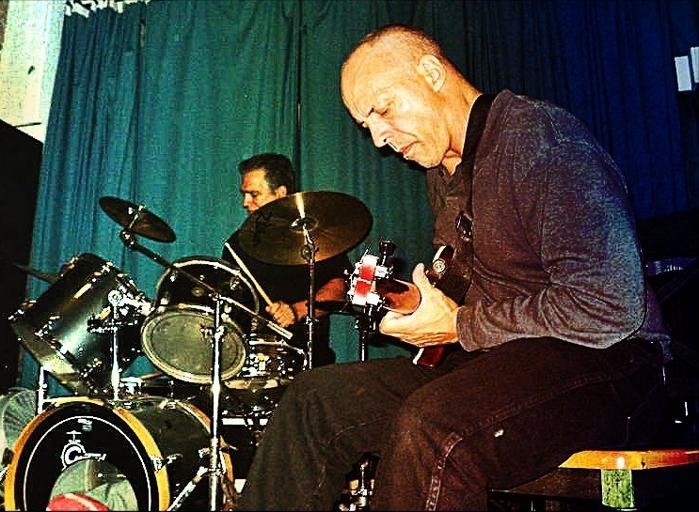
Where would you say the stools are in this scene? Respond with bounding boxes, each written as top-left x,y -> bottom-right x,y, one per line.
545,440 -> 696,512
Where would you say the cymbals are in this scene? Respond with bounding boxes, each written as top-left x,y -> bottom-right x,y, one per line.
100,196 -> 174,244
239,192 -> 372,265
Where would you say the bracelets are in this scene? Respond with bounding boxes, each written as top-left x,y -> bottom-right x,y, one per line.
290,304 -> 298,323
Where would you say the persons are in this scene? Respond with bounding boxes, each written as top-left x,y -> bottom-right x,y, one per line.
219,152 -> 354,364
232,25 -> 674,512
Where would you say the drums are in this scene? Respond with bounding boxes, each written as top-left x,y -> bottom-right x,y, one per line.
4,395 -> 233,512
9,252 -> 153,395
141,256 -> 259,385
213,333 -> 307,426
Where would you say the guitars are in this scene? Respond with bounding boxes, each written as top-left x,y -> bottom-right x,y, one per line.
345,241 -> 457,369
